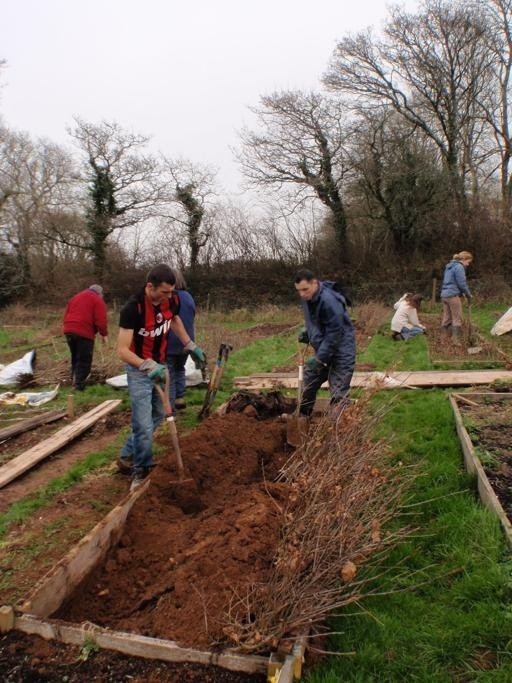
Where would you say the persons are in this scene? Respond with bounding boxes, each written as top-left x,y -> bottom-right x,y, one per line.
441,251 -> 473,345
63,284 -> 108,390
110,265 -> 208,480
294,269 -> 355,423
164,267 -> 195,421
390,293 -> 427,341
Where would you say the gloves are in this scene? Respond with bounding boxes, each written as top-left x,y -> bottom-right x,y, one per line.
183,341 -> 209,370
304,357 -> 325,386
298,329 -> 309,343
139,358 -> 169,384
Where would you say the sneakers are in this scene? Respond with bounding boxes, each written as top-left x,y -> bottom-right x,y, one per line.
281,413 -> 299,423
116,457 -> 134,472
393,334 -> 403,341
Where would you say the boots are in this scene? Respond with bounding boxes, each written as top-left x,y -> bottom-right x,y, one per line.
452,325 -> 471,347
441,325 -> 452,344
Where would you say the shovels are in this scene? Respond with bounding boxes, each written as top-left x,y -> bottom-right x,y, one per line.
152,366 -> 200,514
466,294 -> 481,347
196,343 -> 233,419
287,335 -> 307,448
